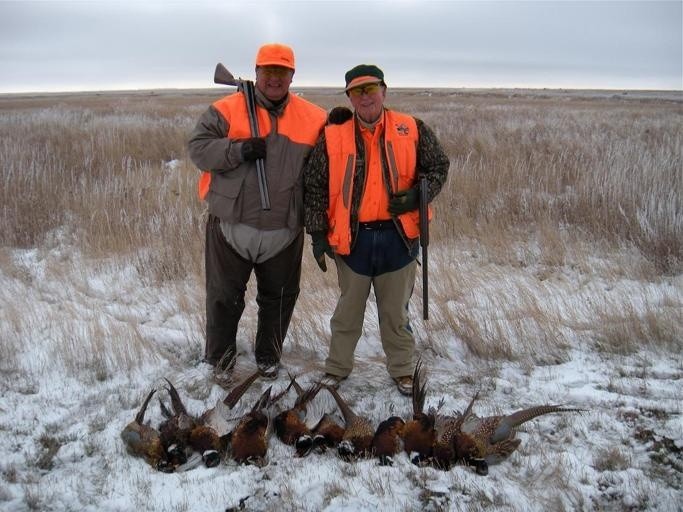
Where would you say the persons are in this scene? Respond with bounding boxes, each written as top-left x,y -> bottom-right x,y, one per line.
301,62 -> 449,395
185,42 -> 353,391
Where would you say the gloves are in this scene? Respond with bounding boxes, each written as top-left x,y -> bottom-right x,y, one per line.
240,137 -> 267,164
387,188 -> 420,217
311,231 -> 335,272
328,107 -> 352,125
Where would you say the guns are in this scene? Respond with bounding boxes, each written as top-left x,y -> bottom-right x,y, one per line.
214,63 -> 271,211
417,172 -> 429,321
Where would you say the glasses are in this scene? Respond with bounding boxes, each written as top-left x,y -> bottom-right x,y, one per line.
348,85 -> 380,97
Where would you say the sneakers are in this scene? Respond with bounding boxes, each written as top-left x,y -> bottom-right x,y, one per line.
211,368 -> 233,387
392,374 -> 414,396
318,372 -> 346,390
256,362 -> 279,381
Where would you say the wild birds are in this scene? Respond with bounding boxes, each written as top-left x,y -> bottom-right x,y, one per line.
120,352 -> 591,476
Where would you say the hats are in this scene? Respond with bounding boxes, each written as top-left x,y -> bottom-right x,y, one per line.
342,63 -> 385,94
255,43 -> 296,70
